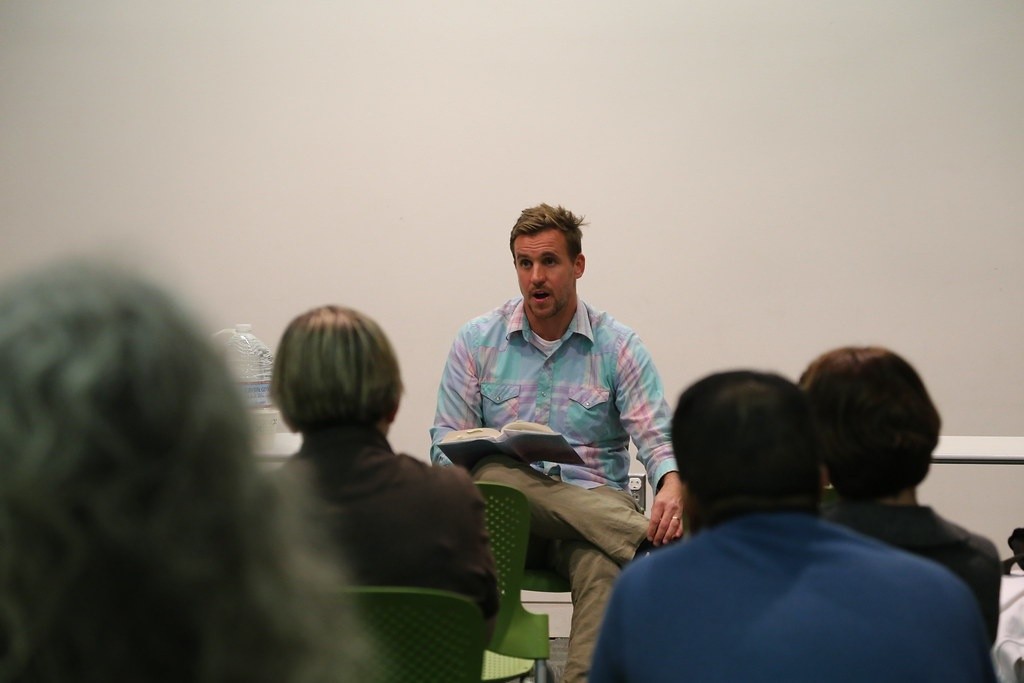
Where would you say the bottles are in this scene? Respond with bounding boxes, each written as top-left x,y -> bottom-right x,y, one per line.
225,323 -> 279,451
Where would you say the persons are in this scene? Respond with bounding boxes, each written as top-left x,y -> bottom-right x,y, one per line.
0,253 -> 501,683
588,347 -> 1002,683
430,201 -> 685,683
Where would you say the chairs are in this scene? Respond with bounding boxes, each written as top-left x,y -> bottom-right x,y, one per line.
326,473 -> 575,683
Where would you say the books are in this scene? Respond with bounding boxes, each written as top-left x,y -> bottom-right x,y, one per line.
436,420 -> 586,473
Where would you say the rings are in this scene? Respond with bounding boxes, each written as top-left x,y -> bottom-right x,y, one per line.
672,516 -> 681,520
